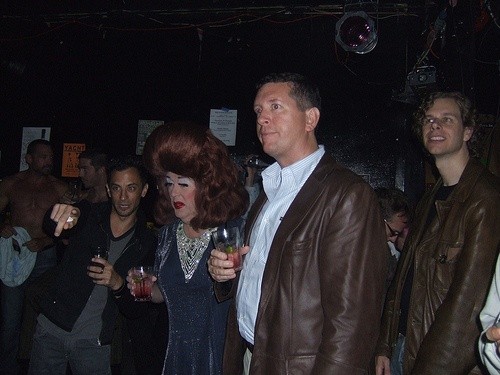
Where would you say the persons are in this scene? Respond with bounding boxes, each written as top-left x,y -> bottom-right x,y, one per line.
78,148 -> 108,202
374,90 -> 500,375
207,73 -> 387,375
126,121 -> 250,375
28,146 -> 148,375
0,139 -> 70,375
375,186 -> 411,244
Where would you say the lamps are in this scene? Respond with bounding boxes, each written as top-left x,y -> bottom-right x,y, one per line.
334,2 -> 378,54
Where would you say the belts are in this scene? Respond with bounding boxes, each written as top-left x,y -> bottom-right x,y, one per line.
246,341 -> 254,353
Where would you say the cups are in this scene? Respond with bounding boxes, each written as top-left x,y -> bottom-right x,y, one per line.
90,242 -> 109,270
129,265 -> 154,302
211,226 -> 246,273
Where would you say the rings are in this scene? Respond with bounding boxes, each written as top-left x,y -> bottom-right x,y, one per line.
100,268 -> 104,274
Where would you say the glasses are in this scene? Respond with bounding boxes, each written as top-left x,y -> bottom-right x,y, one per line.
384,219 -> 400,237
